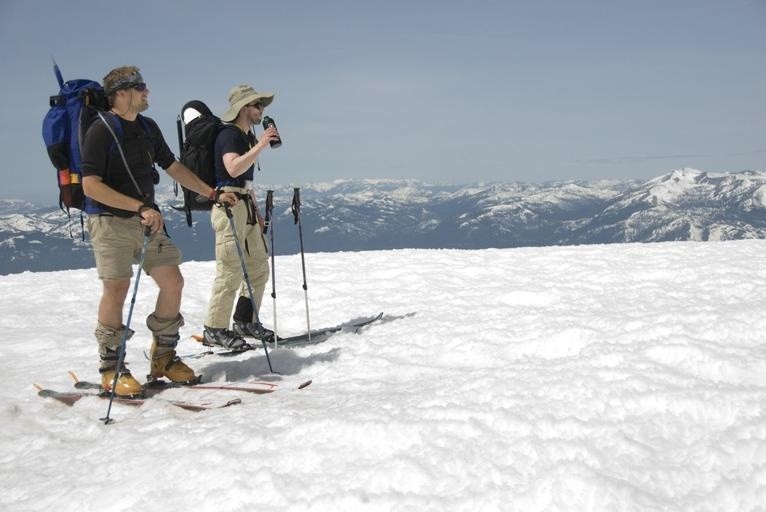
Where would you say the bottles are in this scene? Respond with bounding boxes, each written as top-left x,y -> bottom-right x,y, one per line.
263,116 -> 282,149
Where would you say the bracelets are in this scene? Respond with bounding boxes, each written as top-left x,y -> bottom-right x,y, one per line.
208,189 -> 225,204
136,204 -> 152,220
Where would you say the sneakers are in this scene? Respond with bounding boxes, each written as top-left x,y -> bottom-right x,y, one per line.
151,353 -> 195,382
233,319 -> 274,341
100,366 -> 143,397
201,326 -> 244,348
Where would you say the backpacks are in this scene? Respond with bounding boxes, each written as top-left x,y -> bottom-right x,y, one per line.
40,80 -> 152,209
181,115 -> 258,211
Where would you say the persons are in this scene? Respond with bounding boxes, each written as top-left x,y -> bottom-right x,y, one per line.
81,65 -> 240,398
204,84 -> 280,350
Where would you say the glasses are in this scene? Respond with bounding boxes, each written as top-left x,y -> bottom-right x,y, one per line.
114,82 -> 144,92
247,101 -> 261,108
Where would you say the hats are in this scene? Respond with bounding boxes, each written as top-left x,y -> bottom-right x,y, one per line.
220,85 -> 275,121
